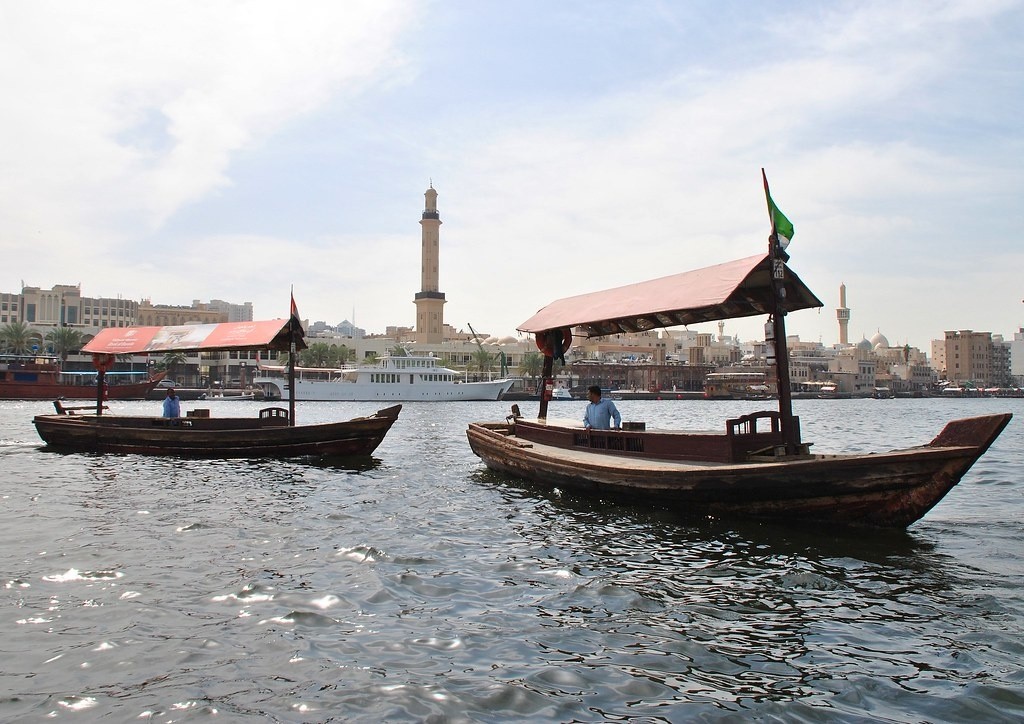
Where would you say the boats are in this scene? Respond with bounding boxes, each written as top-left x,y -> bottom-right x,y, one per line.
465,165 -> 1015,531
32,280 -> 404,462
0,353 -> 170,401
703,322 -> 859,401
252,350 -> 523,402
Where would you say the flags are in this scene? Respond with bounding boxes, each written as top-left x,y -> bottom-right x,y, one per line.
762,171 -> 794,250
291,293 -> 308,349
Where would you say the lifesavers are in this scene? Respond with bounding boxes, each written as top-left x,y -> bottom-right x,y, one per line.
93,353 -> 115,371
536,328 -> 572,357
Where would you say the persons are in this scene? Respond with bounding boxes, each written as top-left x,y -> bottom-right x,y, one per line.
582,386 -> 621,433
163,389 -> 181,418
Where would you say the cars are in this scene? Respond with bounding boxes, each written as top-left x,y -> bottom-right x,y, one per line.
156,379 -> 182,388
